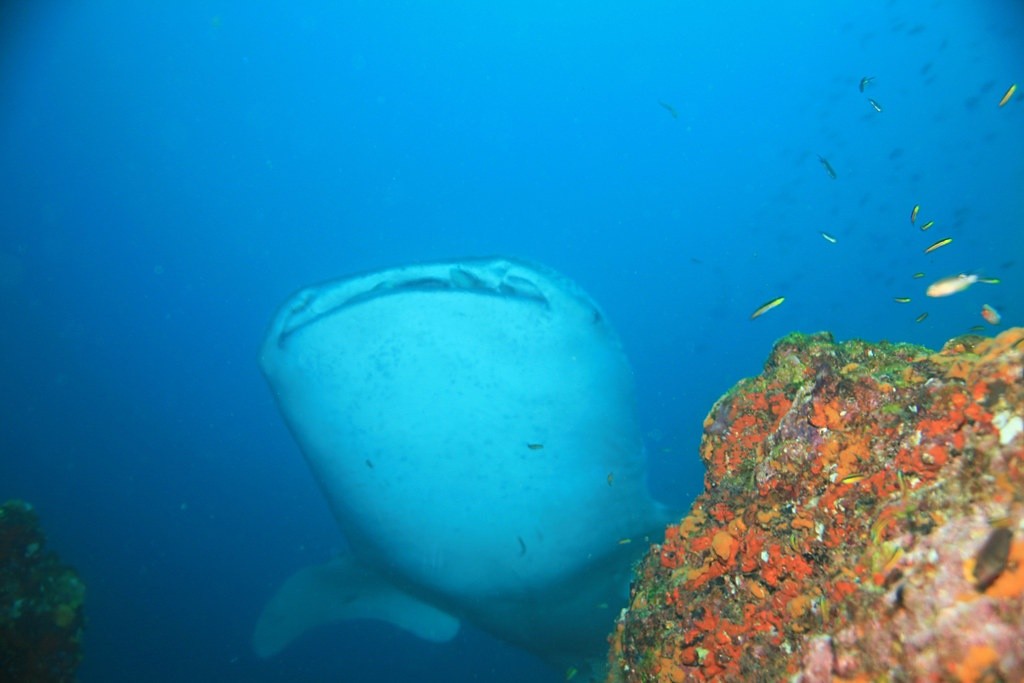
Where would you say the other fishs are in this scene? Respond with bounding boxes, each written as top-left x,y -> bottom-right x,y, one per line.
358,334 -> 1023,682
654,70 -> 1024,334
247,257 -> 683,668
